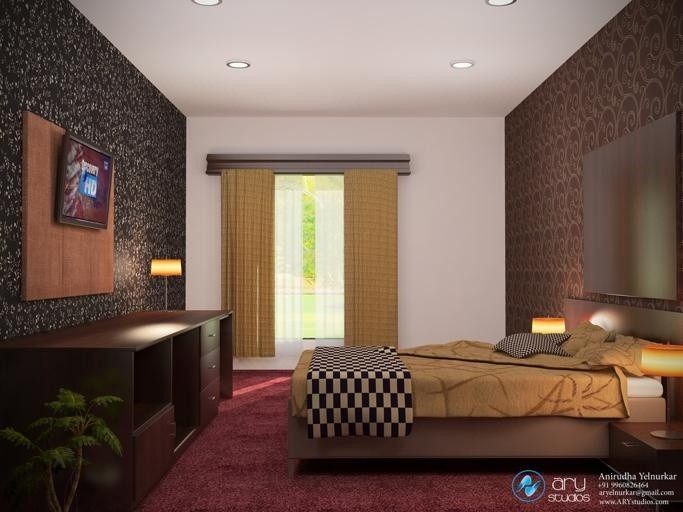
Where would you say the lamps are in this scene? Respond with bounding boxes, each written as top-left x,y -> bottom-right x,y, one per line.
642,342 -> 683,439
151,255 -> 183,311
532,314 -> 567,335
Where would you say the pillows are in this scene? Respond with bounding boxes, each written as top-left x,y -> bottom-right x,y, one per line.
492,319 -> 663,377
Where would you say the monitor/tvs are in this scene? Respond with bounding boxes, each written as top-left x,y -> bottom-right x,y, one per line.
56,128 -> 114,230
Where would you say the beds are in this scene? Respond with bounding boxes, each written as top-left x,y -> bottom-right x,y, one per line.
607,422 -> 683,512
287,298 -> 683,477
